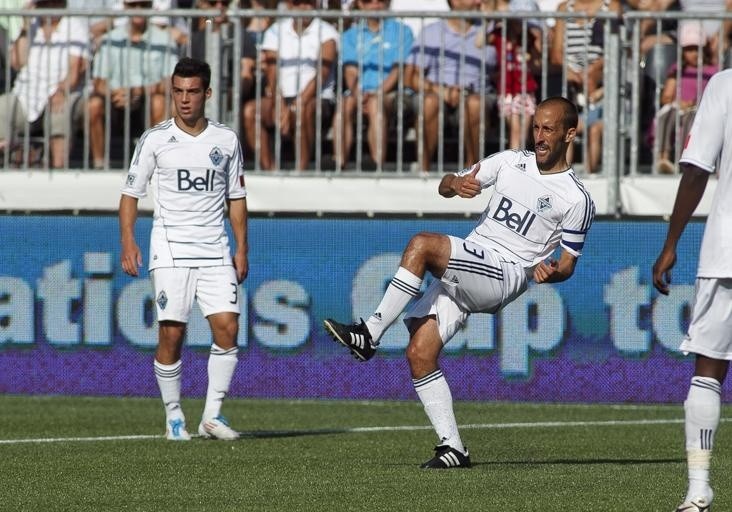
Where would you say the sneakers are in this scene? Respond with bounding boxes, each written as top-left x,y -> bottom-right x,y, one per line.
671,490 -> 716,512
164,415 -> 192,442
419,444 -> 473,469
198,416 -> 241,441
323,316 -> 380,363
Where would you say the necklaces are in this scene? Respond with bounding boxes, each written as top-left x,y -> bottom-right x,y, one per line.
325,98 -> 594,469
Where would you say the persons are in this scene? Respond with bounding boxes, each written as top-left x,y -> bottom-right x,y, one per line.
0,1 -> 732,176
648,62 -> 732,512
118,58 -> 253,443
